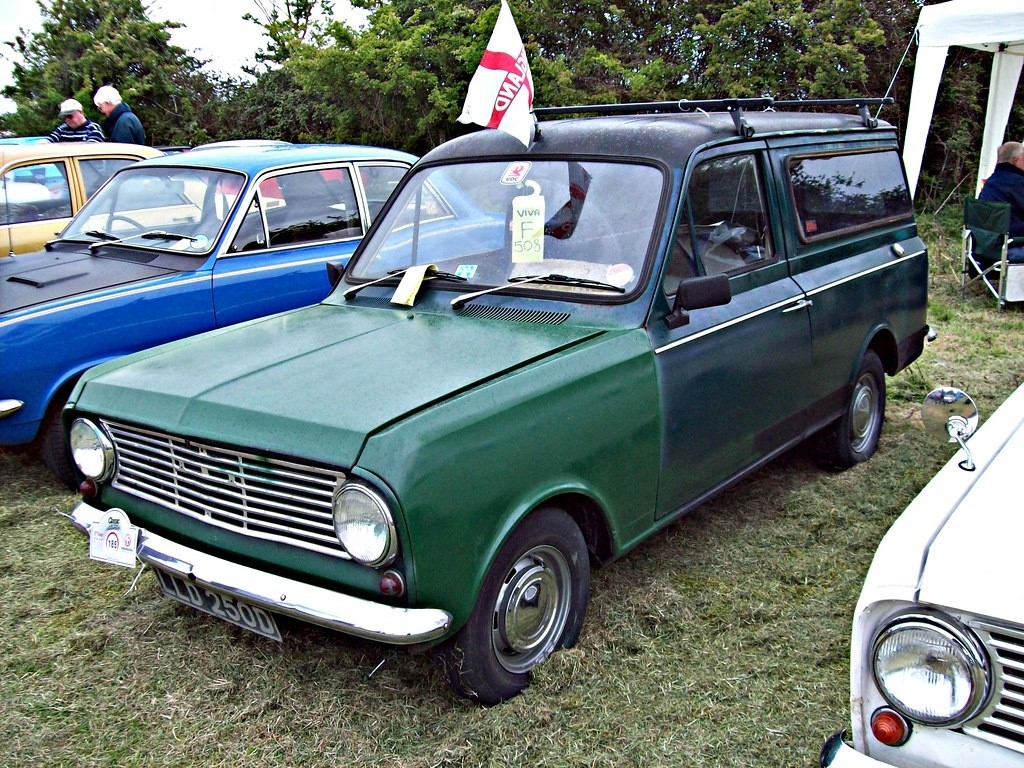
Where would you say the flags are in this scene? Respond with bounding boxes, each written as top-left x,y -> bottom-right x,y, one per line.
455,0 -> 538,149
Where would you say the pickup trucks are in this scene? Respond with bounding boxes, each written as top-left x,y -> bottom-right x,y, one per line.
57,89 -> 936,710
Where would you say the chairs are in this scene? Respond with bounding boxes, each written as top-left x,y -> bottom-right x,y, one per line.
635,233 -> 746,278
957,195 -> 1024,313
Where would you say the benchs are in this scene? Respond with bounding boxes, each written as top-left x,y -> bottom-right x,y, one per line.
285,200 -> 348,237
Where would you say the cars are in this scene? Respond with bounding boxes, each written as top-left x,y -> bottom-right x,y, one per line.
0,144 -> 227,259
0,145 -> 512,494
818,384 -> 1024,768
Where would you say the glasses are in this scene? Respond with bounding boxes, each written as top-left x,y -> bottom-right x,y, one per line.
62,114 -> 73,120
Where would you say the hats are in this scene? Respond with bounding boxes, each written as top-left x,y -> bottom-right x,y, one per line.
58,99 -> 82,118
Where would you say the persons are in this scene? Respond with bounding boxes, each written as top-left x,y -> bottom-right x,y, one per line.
94,85 -> 148,146
37,99 -> 105,142
977,141 -> 1024,264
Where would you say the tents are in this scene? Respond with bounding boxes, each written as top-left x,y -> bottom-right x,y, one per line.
901,0 -> 1023,203
